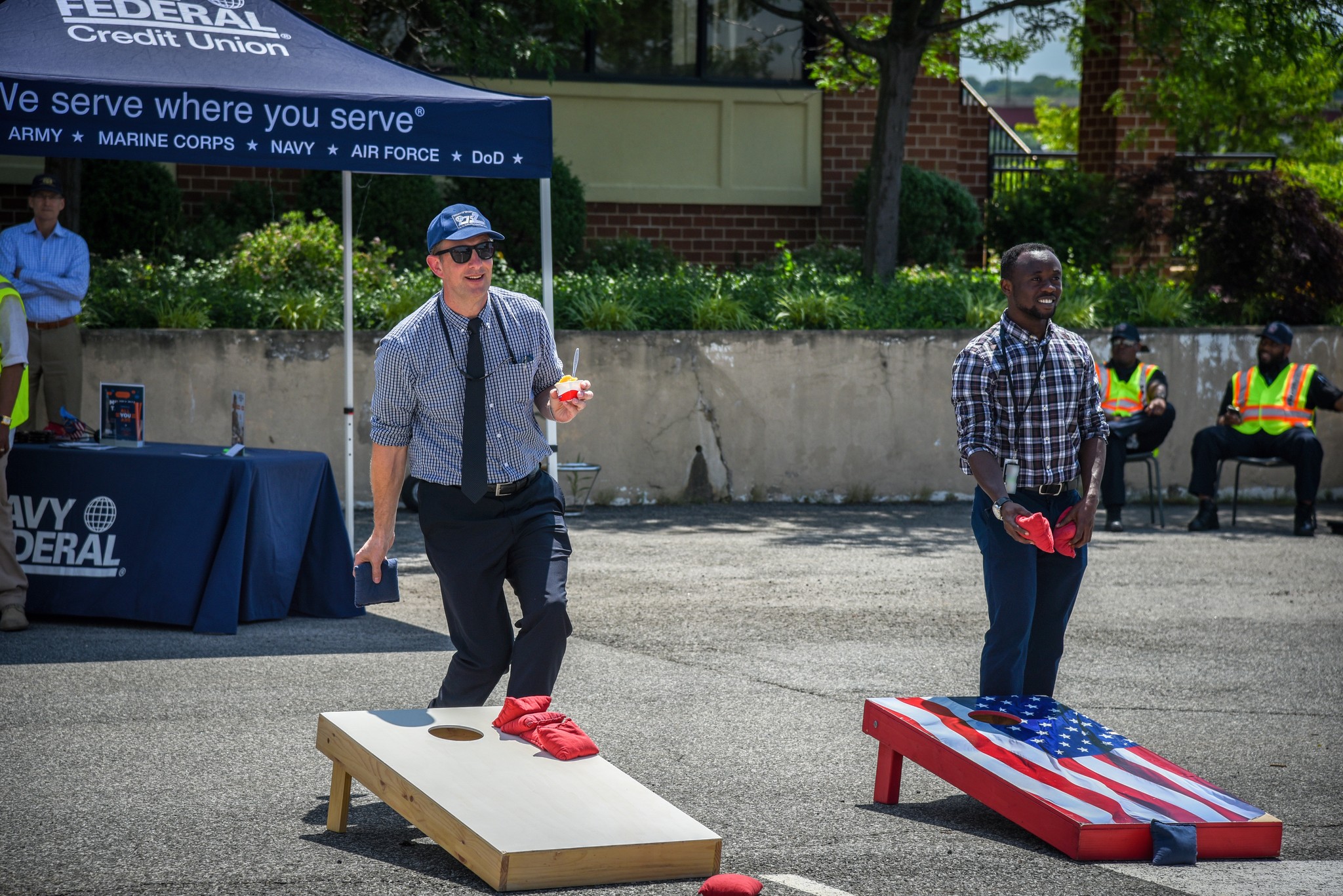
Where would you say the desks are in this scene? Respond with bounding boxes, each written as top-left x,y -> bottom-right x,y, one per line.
5,440 -> 366,635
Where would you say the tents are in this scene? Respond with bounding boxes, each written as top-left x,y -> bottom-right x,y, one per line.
0,0 -> 556,557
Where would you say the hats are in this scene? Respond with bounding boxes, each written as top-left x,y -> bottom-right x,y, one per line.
29,174 -> 66,199
428,204 -> 504,252
1256,322 -> 1293,347
1110,323 -> 1140,343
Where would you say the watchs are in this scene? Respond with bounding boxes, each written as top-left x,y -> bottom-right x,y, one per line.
992,497 -> 1013,521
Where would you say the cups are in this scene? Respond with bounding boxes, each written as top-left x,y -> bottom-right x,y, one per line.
554,380 -> 584,402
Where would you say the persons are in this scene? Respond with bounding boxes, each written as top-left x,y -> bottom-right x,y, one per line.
1092,324 -> 1176,531
1187,321 -> 1343,537
951,242 -> 1110,697
352,204 -> 593,708
0,275 -> 29,630
0,174 -> 90,432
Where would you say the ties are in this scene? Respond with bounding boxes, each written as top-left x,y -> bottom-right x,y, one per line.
460,317 -> 489,505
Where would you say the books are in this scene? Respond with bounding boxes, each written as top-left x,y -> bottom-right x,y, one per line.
51,440 -> 118,451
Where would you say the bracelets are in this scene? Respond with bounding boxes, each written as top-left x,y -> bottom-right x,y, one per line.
549,404 -> 565,423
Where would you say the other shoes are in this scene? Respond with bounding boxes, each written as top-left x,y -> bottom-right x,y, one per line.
1105,504 -> 1125,532
0,604 -> 29,632
1293,508 -> 1317,537
1187,500 -> 1221,532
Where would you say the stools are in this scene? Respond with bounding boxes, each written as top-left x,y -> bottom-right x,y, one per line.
1216,454 -> 1294,527
1125,448 -> 1166,529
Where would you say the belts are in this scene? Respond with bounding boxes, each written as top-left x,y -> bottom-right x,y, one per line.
1022,478 -> 1078,496
25,316 -> 75,331
453,462 -> 545,497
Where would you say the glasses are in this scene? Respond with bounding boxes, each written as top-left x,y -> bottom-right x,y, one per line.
434,242 -> 496,263
1114,339 -> 1138,349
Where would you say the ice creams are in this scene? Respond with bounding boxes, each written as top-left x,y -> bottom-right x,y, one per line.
555,375 -> 578,385
